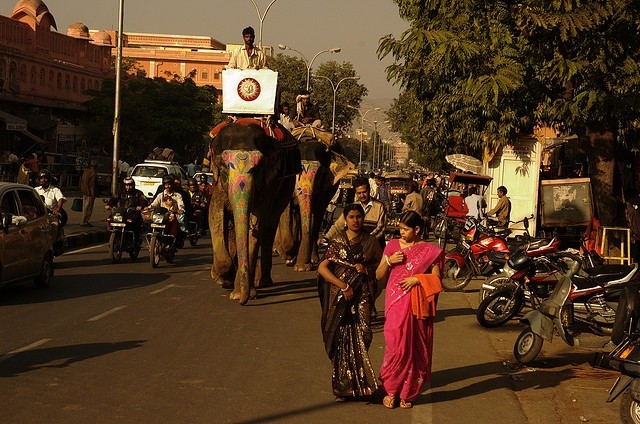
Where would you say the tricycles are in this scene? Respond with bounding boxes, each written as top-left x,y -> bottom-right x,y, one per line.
339,175 -> 356,207
385,175 -> 412,209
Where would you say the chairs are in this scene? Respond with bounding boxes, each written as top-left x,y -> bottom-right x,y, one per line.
446,196 -> 468,217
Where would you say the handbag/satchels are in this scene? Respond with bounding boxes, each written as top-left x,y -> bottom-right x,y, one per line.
71,195 -> 83,212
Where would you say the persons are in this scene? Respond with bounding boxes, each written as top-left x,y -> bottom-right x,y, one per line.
78,162 -> 99,227
399,179 -> 425,213
439,177 -> 448,192
367,171 -> 382,200
10,157 -> 35,184
185,172 -> 201,233
223,27 -> 273,69
485,186 -> 512,227
144,177 -> 187,225
375,211 -> 445,409
315,176 -> 386,246
278,101 -> 295,127
421,171 -> 439,237
463,187 -> 484,226
316,202 -> 382,402
34,169 -> 67,225
291,91 -> 323,128
154,173 -> 185,200
198,174 -> 211,204
117,176 -> 148,221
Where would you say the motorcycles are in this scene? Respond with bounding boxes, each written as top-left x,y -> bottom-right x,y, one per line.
186,213 -> 204,246
476,247 -> 637,328
103,198 -> 144,263
588,312 -> 640,424
513,259 -> 640,364
39,196 -> 65,256
440,218 -> 560,293
145,207 -> 186,269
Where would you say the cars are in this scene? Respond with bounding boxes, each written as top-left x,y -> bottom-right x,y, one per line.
190,172 -> 214,197
0,182 -> 54,294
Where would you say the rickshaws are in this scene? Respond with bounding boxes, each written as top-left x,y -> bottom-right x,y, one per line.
434,173 -> 494,240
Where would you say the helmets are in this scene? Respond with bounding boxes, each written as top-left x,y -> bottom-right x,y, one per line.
122,177 -> 135,194
199,174 -> 208,184
187,178 -> 198,186
37,170 -> 51,187
162,178 -> 175,184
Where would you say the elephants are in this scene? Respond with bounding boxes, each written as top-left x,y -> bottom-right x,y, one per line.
273,125 -> 350,271
207,119 -> 298,305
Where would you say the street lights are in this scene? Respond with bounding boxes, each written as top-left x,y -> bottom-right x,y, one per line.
352,105 -> 401,168
312,74 -> 361,134
278,44 -> 342,92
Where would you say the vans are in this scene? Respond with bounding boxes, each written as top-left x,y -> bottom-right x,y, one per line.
130,158 -> 192,201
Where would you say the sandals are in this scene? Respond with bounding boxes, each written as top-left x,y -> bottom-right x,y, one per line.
335,396 -> 346,403
399,399 -> 412,409
383,395 -> 395,409
